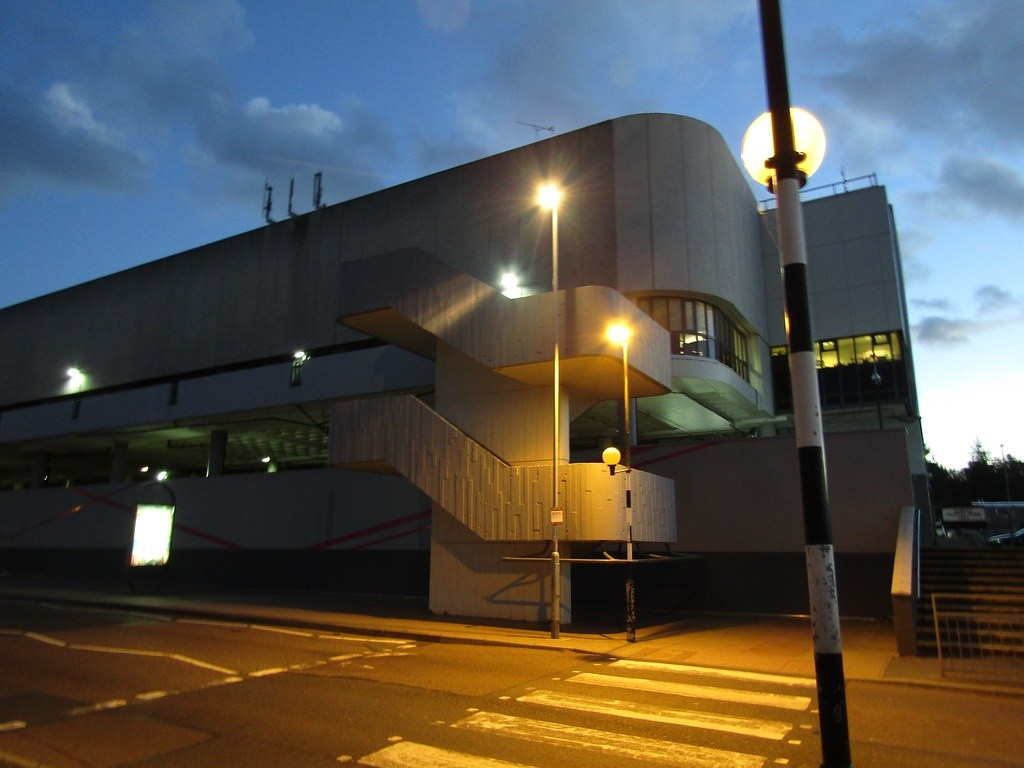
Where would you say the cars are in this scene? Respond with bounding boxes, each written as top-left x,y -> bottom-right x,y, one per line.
987,528 -> 1024,546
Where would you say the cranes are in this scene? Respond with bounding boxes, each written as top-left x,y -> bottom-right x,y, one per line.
514,121 -> 555,144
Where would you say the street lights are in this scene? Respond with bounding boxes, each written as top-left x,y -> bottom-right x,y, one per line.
741,105 -> 870,767
535,179 -> 569,639
600,318 -> 639,646
